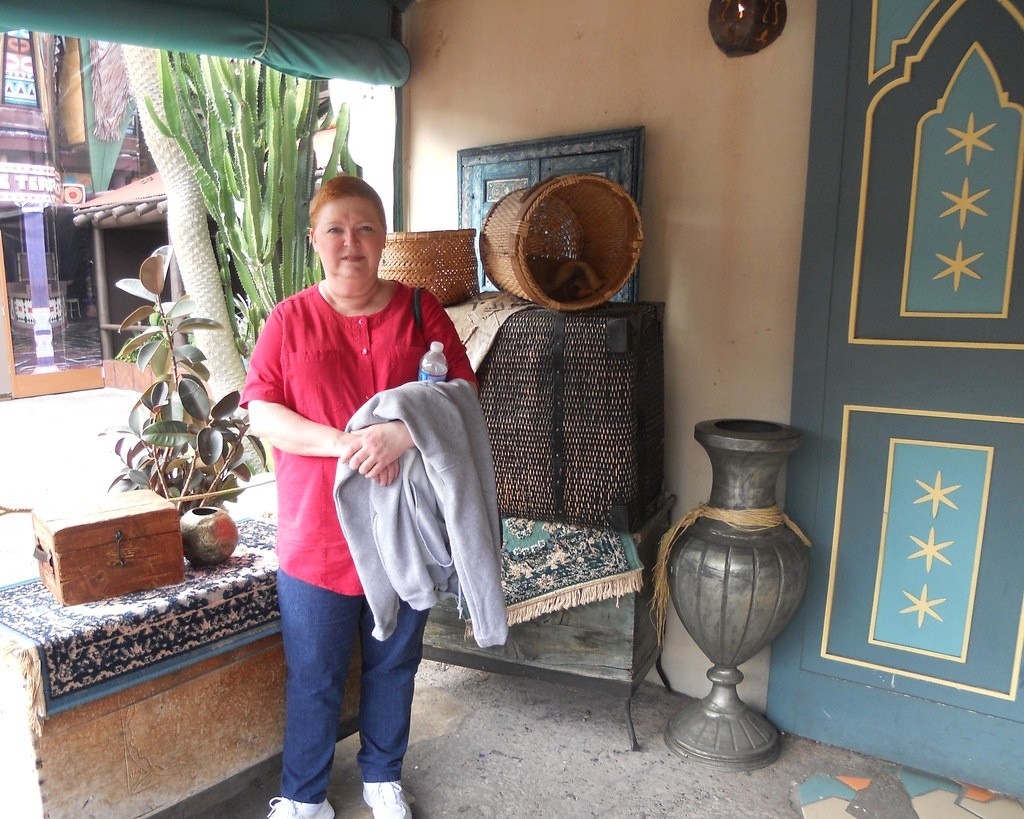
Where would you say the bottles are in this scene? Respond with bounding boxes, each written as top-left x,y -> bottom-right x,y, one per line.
418,341 -> 448,382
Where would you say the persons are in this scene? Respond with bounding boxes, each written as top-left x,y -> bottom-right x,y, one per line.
238,176 -> 481,819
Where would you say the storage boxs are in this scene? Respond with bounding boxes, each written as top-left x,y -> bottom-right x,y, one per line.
27,490 -> 187,608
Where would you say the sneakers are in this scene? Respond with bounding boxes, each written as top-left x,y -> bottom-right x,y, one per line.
364,778 -> 411,818
265,796 -> 336,819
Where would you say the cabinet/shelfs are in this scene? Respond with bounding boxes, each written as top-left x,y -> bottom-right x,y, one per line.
0,511 -> 364,819
432,302 -> 679,752
